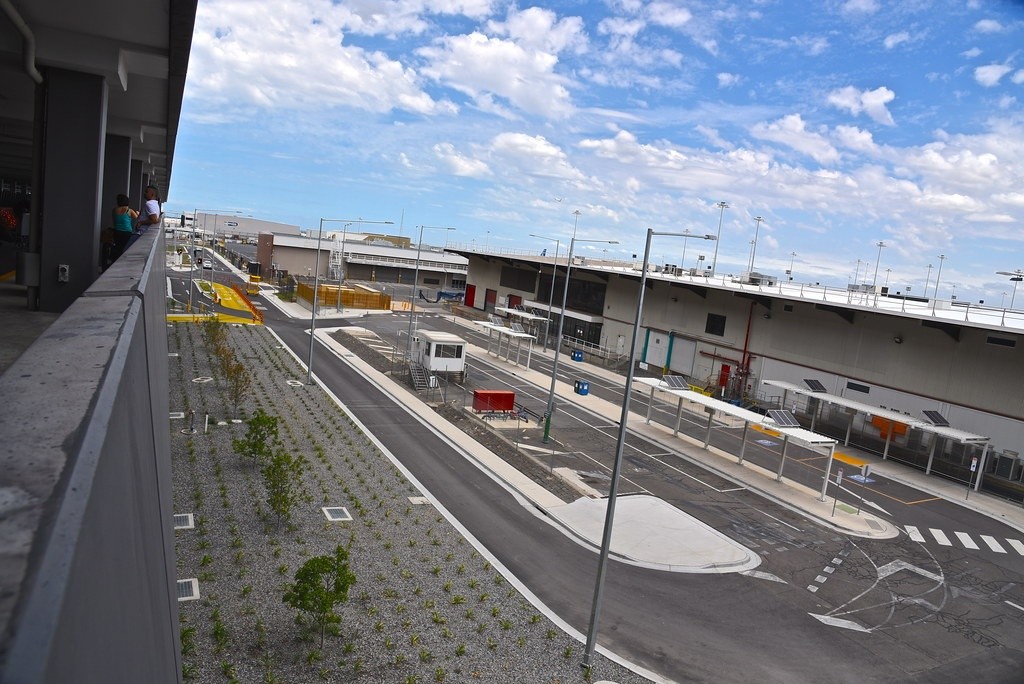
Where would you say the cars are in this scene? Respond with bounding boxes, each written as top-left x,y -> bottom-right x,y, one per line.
204,260 -> 212,270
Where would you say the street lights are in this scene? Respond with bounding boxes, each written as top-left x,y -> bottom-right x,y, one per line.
751,216 -> 766,272
578,228 -> 721,671
680,228 -> 691,270
543,237 -> 619,445
748,240 -> 755,271
1001,292 -> 1007,306
406,225 -> 456,352
712,201 -> 731,274
571,208 -> 582,239
307,218 -> 394,386
529,233 -> 560,352
924,263 -> 935,297
933,253 -> 949,298
873,241 -> 887,286
789,251 -> 797,275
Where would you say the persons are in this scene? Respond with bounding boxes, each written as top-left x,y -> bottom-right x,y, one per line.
123,186 -> 161,251
112,193 -> 140,249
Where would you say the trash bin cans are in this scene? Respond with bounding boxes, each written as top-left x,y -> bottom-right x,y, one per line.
574,379 -> 590,396
570,349 -> 584,362
